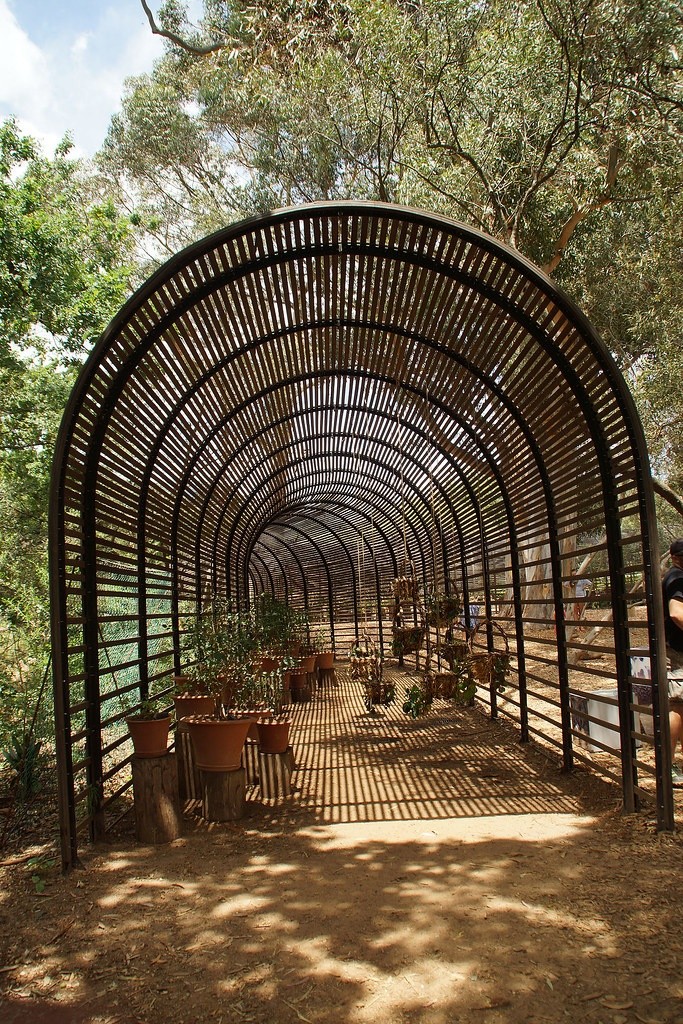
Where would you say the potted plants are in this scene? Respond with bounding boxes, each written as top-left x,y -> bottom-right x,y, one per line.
180,653 -> 252,772
162,618 -> 235,707
467,652 -> 513,693
236,593 -> 315,673
117,682 -> 173,759
402,672 -> 478,719
170,652 -> 204,692
360,679 -> 397,709
256,668 -> 293,754
425,612 -> 452,628
170,668 -> 219,733
389,626 -> 430,657
313,626 -> 336,669
430,596 -> 463,622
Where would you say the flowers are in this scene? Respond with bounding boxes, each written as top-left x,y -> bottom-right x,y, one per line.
283,657 -> 304,673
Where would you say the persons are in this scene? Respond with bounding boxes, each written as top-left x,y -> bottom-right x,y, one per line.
661,538 -> 683,788
461,597 -> 484,642
573,579 -> 592,632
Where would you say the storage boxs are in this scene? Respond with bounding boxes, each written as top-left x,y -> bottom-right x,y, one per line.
569,689 -> 642,753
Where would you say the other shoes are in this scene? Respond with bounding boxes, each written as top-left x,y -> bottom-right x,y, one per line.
671,761 -> 683,787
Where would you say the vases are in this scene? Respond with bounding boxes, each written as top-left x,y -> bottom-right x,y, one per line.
289,671 -> 307,689
